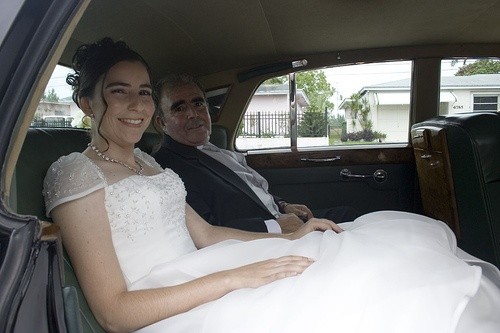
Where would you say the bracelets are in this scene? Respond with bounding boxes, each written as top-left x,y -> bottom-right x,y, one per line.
281,203 -> 291,213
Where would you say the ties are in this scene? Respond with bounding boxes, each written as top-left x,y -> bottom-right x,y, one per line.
203,144 -> 239,162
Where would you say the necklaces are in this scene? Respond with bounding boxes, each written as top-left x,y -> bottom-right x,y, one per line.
87,143 -> 144,176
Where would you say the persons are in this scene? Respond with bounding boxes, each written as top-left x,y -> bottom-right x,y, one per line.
152,75 -> 313,235
45,36 -> 500,333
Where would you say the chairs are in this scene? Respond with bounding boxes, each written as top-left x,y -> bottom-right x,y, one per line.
410,111 -> 500,270
17,124 -> 226,333
